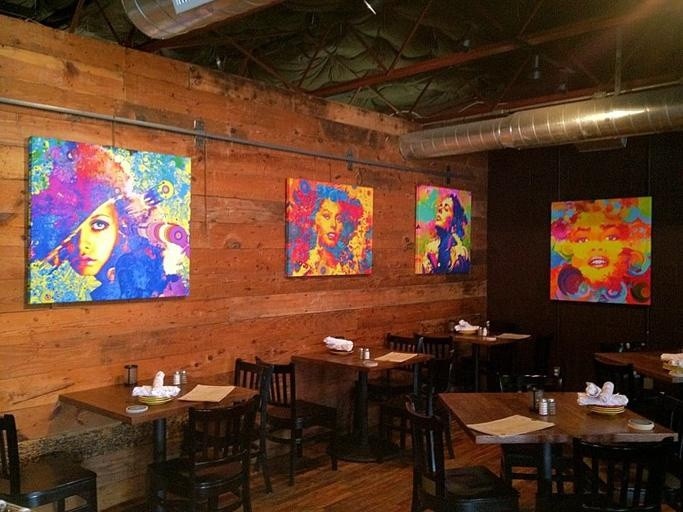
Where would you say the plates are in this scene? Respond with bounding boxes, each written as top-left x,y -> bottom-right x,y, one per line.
663,360 -> 683,377
134,393 -> 175,405
589,405 -> 626,415
329,350 -> 354,355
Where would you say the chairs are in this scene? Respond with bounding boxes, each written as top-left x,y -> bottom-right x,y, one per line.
255,356 -> 338,486
653,391 -> 683,511
147,395 -> 261,511
181,358 -> 274,494
596,337 -> 661,404
1,413 -> 98,512
354,332 -> 456,459
404,393 -> 521,511
492,365 -> 563,495
535,436 -> 674,512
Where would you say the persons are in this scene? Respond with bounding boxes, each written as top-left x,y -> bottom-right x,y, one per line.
29,144 -> 188,300
293,190 -> 360,277
551,200 -> 652,305
422,193 -> 472,274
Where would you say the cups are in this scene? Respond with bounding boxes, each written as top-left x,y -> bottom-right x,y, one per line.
527,384 -> 545,412
124,364 -> 137,386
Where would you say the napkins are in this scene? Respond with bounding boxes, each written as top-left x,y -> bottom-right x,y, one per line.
178,384 -> 236,403
494,333 -> 532,339
465,414 -> 555,438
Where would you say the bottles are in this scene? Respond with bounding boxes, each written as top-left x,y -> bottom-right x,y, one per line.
538,398 -> 557,415
172,369 -> 189,386
359,348 -> 370,360
447,319 -> 491,336
